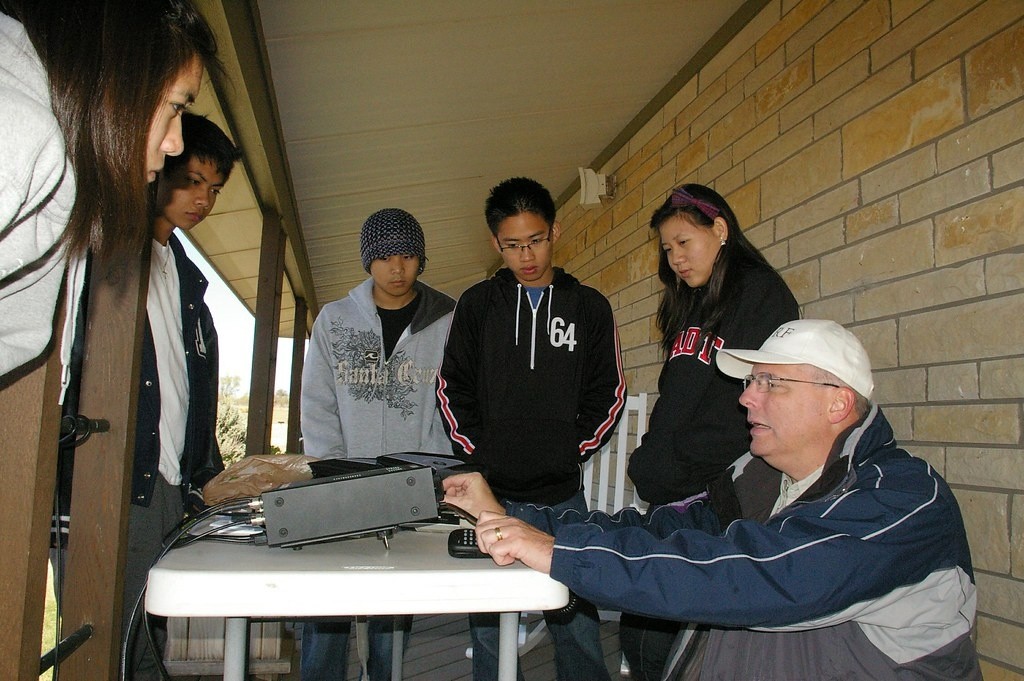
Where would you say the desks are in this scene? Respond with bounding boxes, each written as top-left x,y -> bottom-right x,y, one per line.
146,529 -> 569,681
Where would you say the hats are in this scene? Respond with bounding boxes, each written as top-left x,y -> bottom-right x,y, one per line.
716,318 -> 875,404
359,208 -> 425,276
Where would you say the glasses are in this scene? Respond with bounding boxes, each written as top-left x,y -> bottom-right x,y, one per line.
496,225 -> 551,255
743,372 -> 841,393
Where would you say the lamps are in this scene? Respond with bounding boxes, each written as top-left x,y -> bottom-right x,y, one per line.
577,166 -> 617,210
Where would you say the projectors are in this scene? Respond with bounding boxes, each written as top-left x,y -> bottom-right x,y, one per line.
262,463 -> 439,548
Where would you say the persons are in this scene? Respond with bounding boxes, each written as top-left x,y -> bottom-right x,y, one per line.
618,183 -> 799,681
0,0 -> 218,389
441,319 -> 985,681
434,177 -> 629,681
298,207 -> 457,681
47,112 -> 239,681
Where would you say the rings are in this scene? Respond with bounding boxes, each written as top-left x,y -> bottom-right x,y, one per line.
495,528 -> 502,541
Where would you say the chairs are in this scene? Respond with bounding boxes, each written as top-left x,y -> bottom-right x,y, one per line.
464,392 -> 648,660
